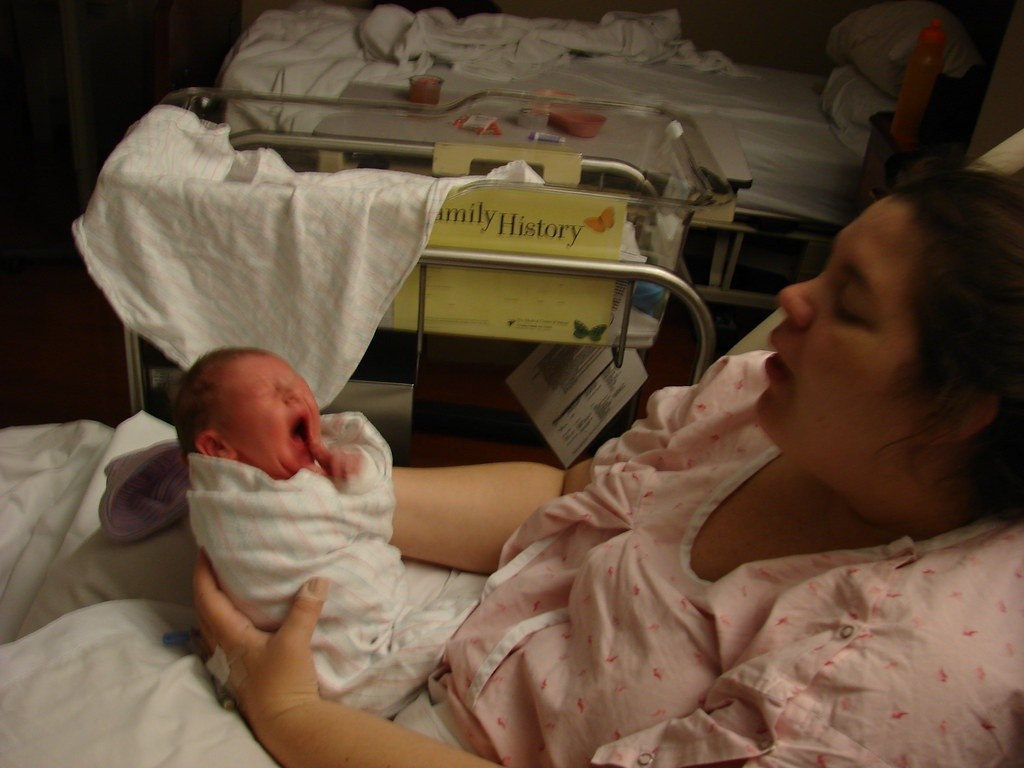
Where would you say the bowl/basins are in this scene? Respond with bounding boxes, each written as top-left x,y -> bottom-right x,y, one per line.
565,112 -> 607,137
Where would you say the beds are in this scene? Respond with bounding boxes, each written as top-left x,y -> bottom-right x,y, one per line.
213,0 -> 1024,310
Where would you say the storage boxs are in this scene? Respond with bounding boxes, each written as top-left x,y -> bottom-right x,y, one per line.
375,183 -> 627,347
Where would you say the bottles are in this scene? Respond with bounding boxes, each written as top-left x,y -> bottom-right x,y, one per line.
890,18 -> 948,149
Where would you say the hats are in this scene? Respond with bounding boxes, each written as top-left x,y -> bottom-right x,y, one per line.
98,440 -> 193,541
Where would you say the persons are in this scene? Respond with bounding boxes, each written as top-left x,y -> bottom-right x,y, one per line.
191,161 -> 1024,768
173,346 -> 439,694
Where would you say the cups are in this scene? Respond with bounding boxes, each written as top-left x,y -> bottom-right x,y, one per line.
521,91 -> 550,130
409,75 -> 445,105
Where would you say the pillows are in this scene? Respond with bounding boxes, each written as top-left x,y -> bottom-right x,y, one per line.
816,61 -> 957,158
821,3 -> 984,102
